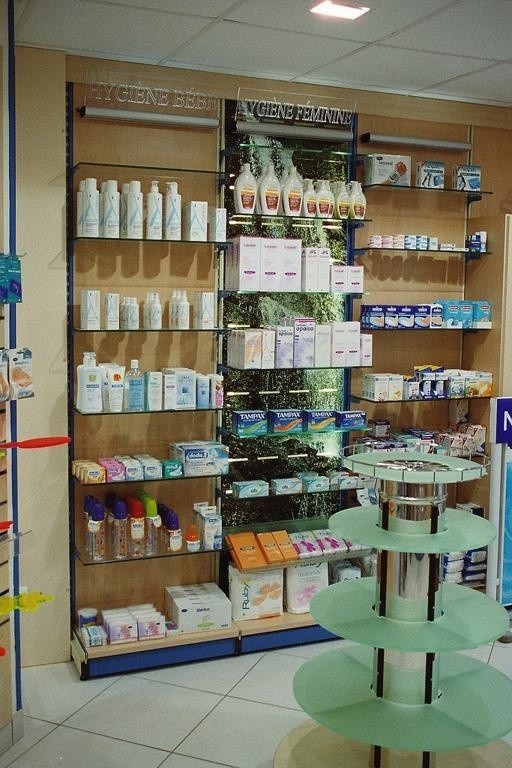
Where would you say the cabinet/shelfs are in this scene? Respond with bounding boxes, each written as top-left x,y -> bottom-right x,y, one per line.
64,79 -> 238,682
272,441 -> 512,768
350,132 -> 492,591
221,93 -> 373,655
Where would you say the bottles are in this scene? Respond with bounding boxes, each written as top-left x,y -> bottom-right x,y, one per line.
82,489 -> 183,562
125,360 -> 145,410
